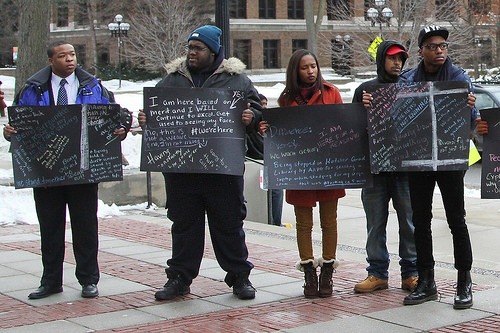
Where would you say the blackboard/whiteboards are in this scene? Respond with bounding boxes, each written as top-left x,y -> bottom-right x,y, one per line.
262,102 -> 374,189
364,81 -> 471,174
139,87 -> 248,176
480,108 -> 500,199
7,104 -> 123,190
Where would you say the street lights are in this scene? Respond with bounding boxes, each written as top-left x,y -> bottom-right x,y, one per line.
367,0 -> 393,39
108,14 -> 130,87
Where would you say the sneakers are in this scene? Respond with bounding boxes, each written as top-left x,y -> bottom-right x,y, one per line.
402,275 -> 419,293
354,275 -> 388,293
233,283 -> 257,300
155,277 -> 191,300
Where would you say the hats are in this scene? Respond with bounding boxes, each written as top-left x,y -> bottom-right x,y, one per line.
386,45 -> 406,55
188,25 -> 222,55
418,25 -> 449,49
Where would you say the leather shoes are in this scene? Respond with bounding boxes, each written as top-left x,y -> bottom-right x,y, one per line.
28,285 -> 63,299
81,284 -> 100,298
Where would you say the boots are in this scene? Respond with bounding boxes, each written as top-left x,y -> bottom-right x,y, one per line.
319,257 -> 340,298
295,255 -> 318,299
453,270 -> 473,309
403,268 -> 438,305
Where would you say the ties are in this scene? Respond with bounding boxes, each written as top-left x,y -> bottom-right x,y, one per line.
57,79 -> 69,105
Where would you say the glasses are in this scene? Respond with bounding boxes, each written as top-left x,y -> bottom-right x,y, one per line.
184,45 -> 208,53
421,42 -> 449,51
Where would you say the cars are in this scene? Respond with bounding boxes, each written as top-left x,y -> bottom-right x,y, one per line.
472,80 -> 500,150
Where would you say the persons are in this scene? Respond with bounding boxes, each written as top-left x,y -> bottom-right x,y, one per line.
2,42 -> 132,298
246,93 -> 284,226
258,48 -> 345,299
137,25 -> 263,301
475,117 -> 488,135
353,40 -> 419,293
363,25 -> 477,309
